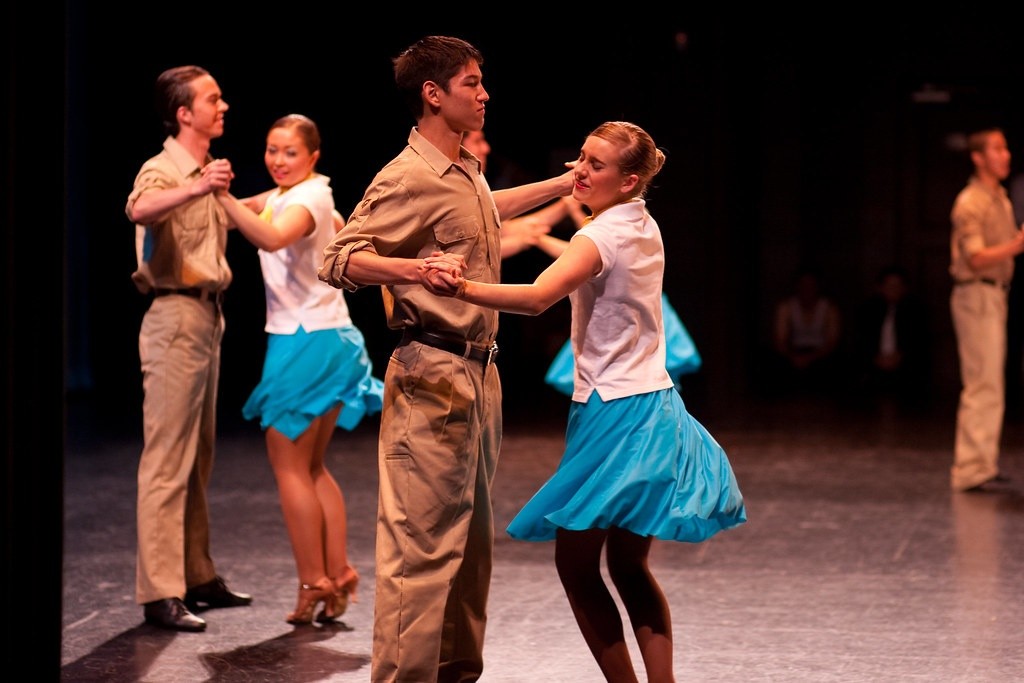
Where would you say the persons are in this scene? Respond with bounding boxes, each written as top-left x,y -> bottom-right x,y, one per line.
319,35 -> 746,683
860,263 -> 935,416
947,129 -> 1024,496
125,64 -> 253,633
774,272 -> 839,392
198,112 -> 386,623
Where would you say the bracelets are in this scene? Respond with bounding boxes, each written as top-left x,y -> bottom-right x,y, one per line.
577,216 -> 591,228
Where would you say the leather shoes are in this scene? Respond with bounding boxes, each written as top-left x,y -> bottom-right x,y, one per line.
184,576 -> 252,607
144,597 -> 207,632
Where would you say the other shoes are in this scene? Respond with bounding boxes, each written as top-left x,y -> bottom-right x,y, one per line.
966,480 -> 1011,494
987,473 -> 1012,483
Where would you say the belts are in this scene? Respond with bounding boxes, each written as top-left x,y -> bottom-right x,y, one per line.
982,277 -> 1011,291
404,326 -> 499,364
154,286 -> 224,303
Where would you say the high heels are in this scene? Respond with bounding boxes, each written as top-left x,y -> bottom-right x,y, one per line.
286,576 -> 340,625
317,567 -> 360,623
